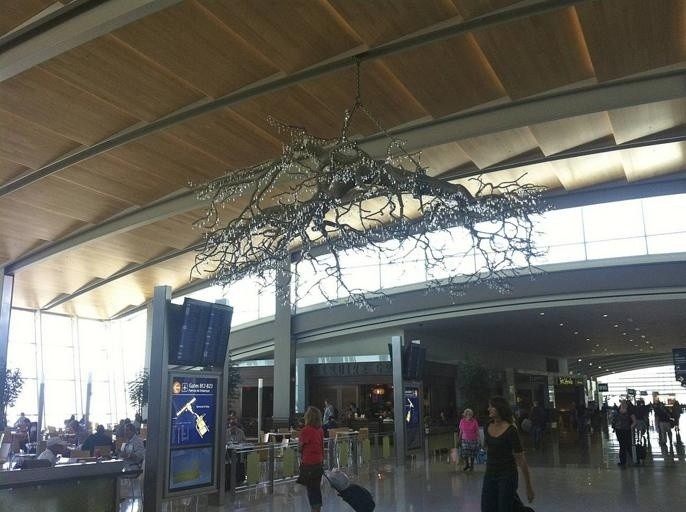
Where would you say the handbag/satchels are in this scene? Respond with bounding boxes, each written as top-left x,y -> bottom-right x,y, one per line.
296,473 -> 321,488
327,468 -> 350,491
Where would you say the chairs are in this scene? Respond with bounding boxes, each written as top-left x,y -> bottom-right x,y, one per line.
0,424 -> 147,502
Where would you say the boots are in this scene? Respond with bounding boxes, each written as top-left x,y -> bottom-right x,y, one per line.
469,456 -> 474,471
463,456 -> 470,471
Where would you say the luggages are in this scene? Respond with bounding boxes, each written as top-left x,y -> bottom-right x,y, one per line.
320,467 -> 375,512
631,428 -> 639,464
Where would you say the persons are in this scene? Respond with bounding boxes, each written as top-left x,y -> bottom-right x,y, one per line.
7,413 -> 146,504
224,384 -> 684,474
479,394 -> 536,512
298,408 -> 326,512
